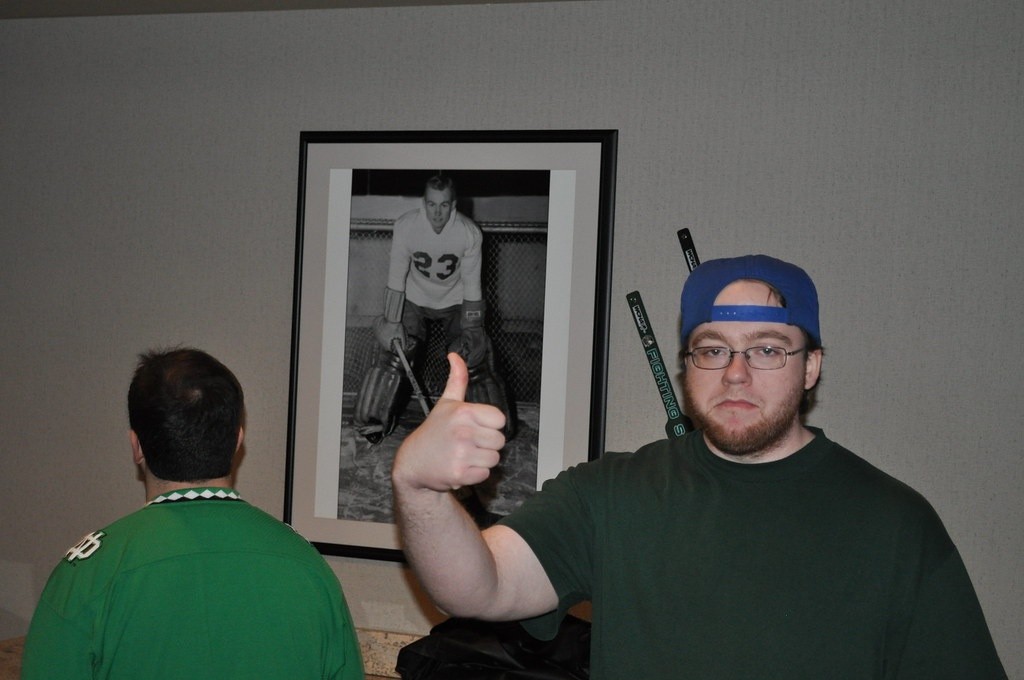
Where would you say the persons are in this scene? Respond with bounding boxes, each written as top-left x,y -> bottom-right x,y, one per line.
20,349 -> 368,680
391,255 -> 1007,680
354,175 -> 512,443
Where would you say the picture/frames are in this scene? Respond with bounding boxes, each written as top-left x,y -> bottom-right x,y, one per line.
284,129 -> 619,562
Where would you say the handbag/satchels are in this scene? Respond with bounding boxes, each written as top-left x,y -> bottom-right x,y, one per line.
395,611 -> 591,680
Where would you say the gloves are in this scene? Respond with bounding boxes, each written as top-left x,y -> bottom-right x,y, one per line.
461,299 -> 489,367
375,286 -> 408,353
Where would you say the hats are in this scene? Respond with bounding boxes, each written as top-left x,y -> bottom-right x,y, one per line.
681,254 -> 821,347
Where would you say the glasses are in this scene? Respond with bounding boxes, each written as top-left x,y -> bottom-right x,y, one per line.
683,346 -> 807,370
